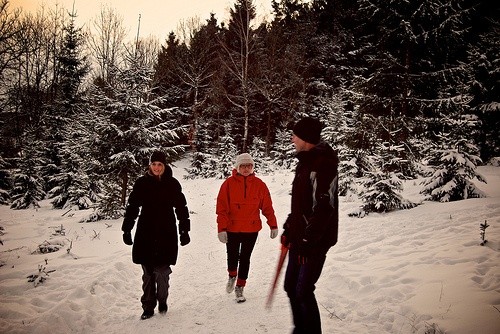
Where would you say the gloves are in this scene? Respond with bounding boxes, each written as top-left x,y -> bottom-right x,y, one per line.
179,232 -> 190,246
123,231 -> 133,245
218,231 -> 228,244
270,229 -> 278,239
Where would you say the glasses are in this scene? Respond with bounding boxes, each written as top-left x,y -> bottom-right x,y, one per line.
241,163 -> 252,169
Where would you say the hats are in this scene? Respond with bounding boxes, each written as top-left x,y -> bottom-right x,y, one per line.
150,151 -> 167,166
235,153 -> 255,170
294,117 -> 327,144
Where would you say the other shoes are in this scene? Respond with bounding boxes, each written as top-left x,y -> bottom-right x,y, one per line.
158,296 -> 168,313
141,309 -> 154,319
225,273 -> 237,293
235,286 -> 246,303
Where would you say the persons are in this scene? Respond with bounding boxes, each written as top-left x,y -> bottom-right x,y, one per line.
281,117 -> 339,334
216,153 -> 279,303
121,151 -> 190,319
